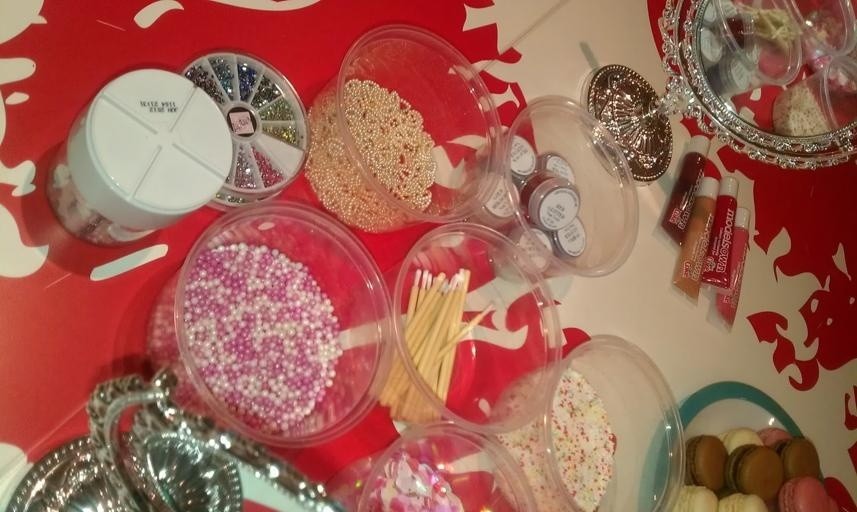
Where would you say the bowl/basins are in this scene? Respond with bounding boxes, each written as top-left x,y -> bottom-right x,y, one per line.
143,21 -> 683,508
697,1 -> 854,138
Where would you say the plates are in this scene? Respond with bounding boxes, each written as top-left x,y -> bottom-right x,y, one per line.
0,428 -> 339,512
638,378 -> 828,510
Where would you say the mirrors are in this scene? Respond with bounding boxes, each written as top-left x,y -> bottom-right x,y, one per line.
6,364 -> 348,512
581,0 -> 857,189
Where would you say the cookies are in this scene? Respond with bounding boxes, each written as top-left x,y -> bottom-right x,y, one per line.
667,426 -> 838,512
